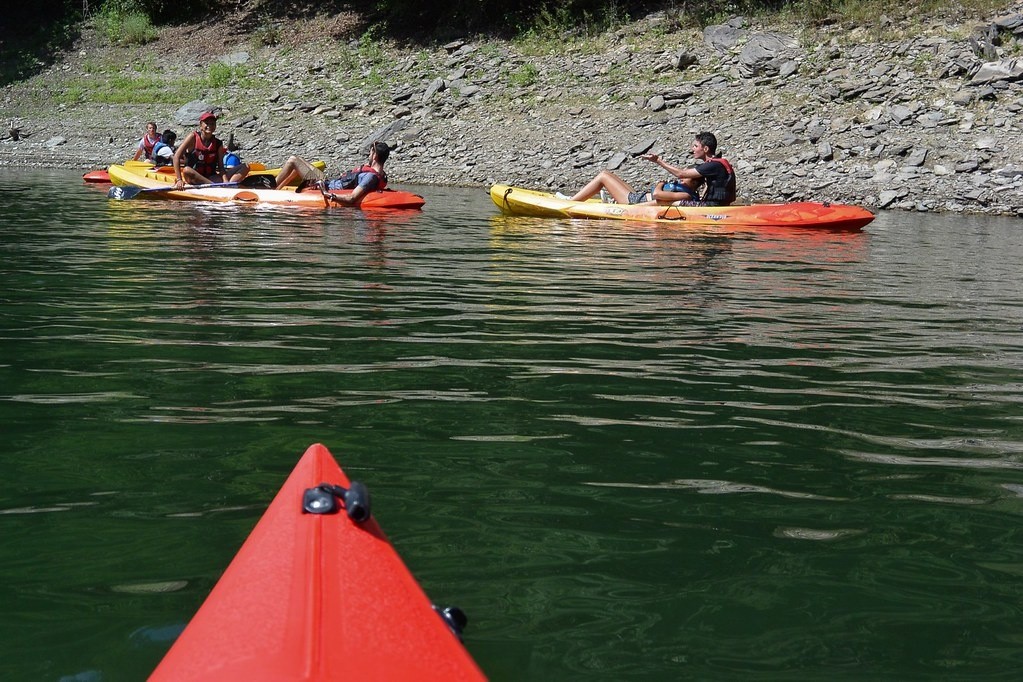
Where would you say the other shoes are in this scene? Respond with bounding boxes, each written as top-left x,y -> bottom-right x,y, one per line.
556,191 -> 572,202
260,175 -> 275,189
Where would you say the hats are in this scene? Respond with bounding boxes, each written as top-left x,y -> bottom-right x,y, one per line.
199,112 -> 218,121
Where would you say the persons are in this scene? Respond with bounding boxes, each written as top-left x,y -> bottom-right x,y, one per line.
175,113 -> 250,192
150,130 -> 184,167
133,122 -> 164,162
276,141 -> 390,208
216,153 -> 241,168
556,164 -> 704,205
634,131 -> 736,207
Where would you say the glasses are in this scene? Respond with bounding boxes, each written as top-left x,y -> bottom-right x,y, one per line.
373,140 -> 379,153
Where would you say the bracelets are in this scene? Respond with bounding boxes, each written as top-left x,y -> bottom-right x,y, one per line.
176,178 -> 182,181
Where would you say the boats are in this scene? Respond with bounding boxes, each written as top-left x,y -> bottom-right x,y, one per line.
124,160 -> 326,183
83,169 -> 111,182
491,181 -> 875,232
108,164 -> 425,210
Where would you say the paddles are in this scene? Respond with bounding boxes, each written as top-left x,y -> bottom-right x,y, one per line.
108,174 -> 277,200
295,180 -> 330,193
